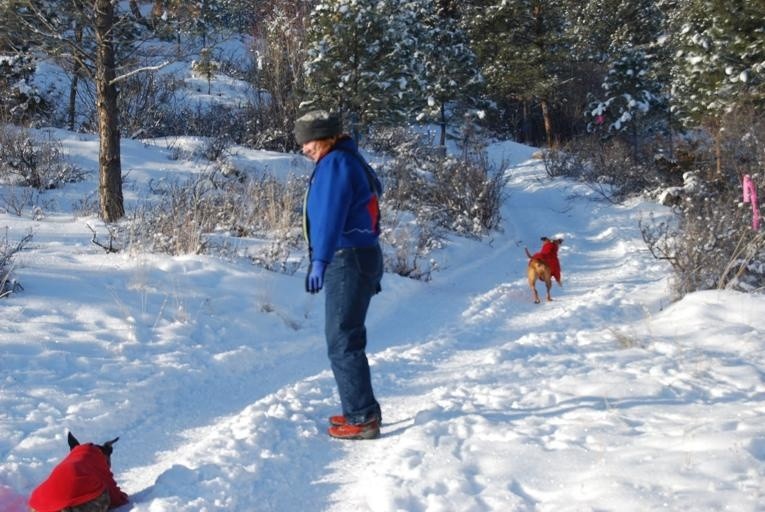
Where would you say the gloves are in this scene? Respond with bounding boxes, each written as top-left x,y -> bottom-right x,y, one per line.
305,260 -> 328,294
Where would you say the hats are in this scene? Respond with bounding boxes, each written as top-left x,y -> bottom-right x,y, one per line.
293,109 -> 341,145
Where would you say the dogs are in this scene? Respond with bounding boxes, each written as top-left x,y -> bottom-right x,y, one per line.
523,234 -> 565,302
28,430 -> 128,512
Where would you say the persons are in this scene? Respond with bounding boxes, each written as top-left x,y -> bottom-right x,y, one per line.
292,108 -> 386,442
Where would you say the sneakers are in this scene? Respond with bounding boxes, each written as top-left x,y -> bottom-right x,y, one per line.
329,415 -> 346,426
327,421 -> 380,439
